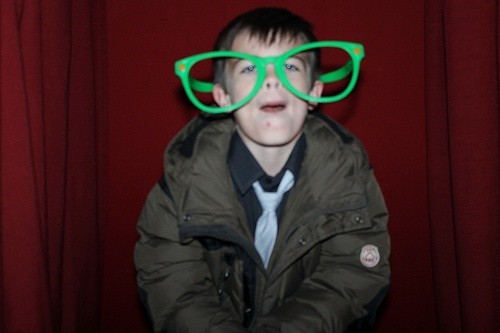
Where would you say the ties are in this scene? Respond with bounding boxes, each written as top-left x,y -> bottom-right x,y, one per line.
252,170 -> 295,269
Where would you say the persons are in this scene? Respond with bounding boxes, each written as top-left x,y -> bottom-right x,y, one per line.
133,6 -> 392,333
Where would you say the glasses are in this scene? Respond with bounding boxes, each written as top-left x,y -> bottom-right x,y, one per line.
174,40 -> 365,114
